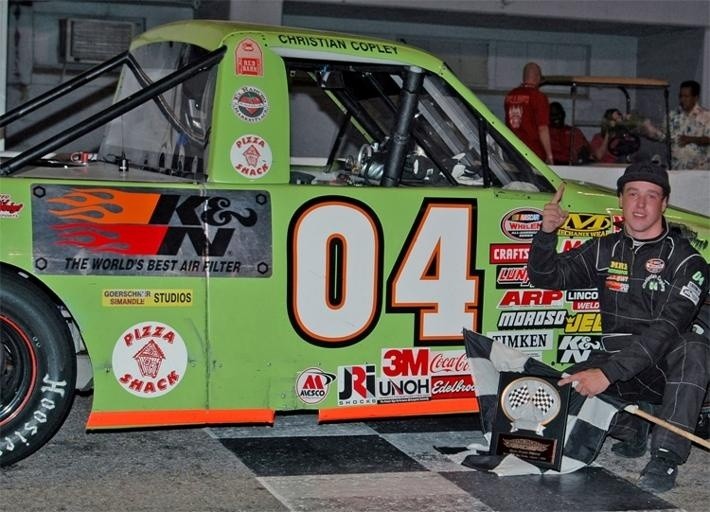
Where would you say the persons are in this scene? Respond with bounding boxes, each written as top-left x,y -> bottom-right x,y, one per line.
504,62 -> 710,170
526,162 -> 710,493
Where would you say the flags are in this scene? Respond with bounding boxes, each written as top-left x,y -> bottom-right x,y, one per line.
531,384 -> 555,414
460,328 -> 639,474
506,385 -> 530,411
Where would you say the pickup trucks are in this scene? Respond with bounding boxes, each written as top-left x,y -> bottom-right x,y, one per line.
0,18 -> 710,471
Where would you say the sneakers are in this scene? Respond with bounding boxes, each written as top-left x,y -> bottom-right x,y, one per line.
611,400 -> 653,459
637,456 -> 679,491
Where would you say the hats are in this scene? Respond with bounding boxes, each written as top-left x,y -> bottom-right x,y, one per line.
617,161 -> 671,196
